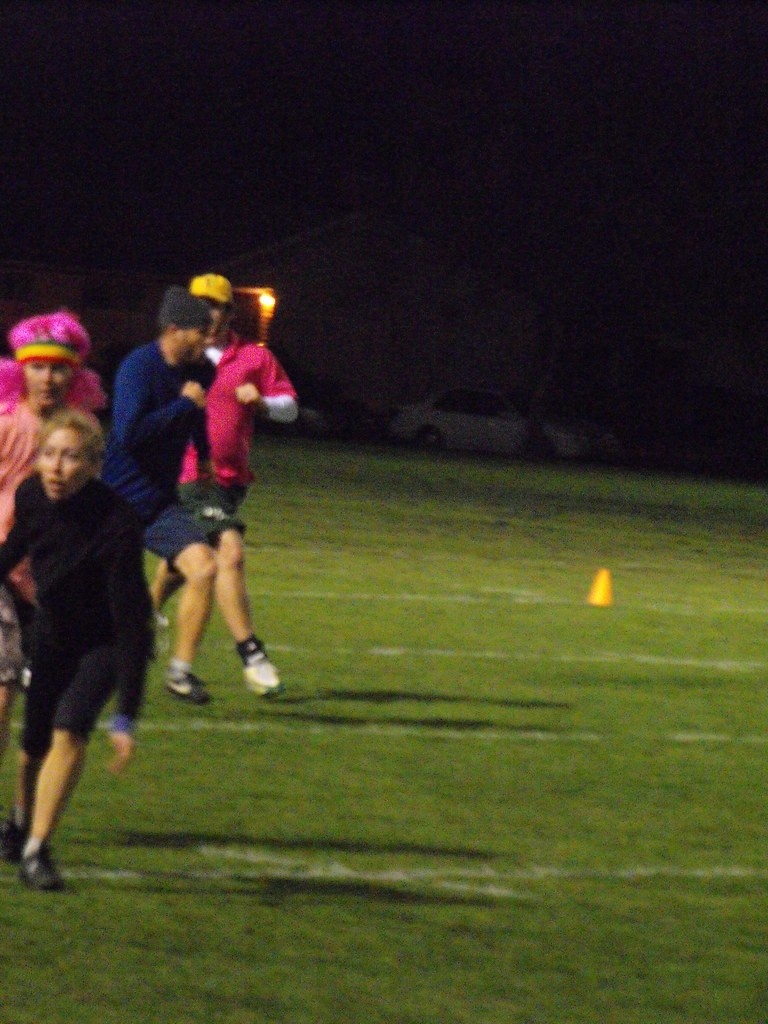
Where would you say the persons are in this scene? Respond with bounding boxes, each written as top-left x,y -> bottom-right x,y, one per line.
101,274 -> 298,703
0,411 -> 151,890
0,306 -> 104,833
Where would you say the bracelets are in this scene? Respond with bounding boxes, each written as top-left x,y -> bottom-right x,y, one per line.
113,713 -> 134,734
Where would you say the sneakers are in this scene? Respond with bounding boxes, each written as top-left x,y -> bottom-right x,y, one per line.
155,612 -> 169,628
235,635 -> 286,696
160,670 -> 214,707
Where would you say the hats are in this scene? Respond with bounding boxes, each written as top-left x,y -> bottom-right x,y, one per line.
191,273 -> 236,303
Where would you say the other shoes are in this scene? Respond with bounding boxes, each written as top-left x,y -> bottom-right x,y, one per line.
21,847 -> 63,894
0,821 -> 31,862
